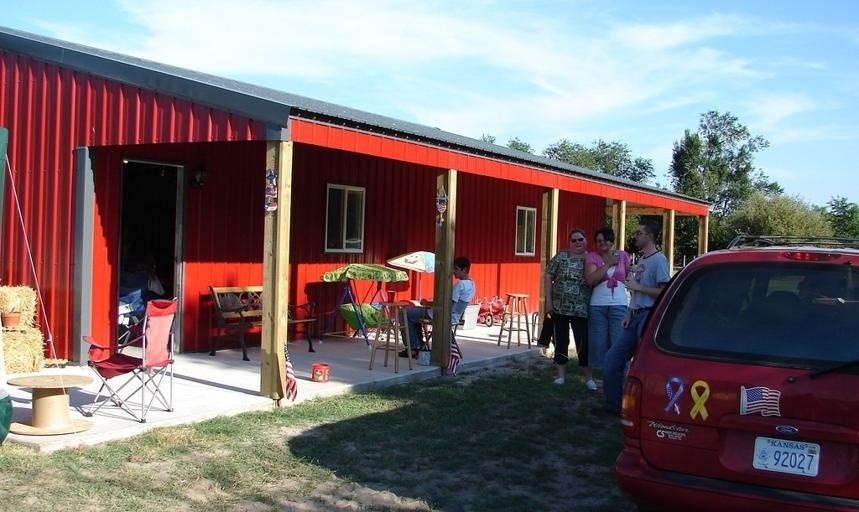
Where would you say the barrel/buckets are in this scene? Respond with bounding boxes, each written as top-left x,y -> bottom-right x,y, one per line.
312,364 -> 329,381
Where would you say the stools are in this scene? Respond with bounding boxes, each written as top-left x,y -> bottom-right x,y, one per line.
370,301 -> 413,374
5,375 -> 92,436
498,293 -> 532,349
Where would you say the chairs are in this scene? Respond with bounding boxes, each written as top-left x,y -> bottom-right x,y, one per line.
412,299 -> 469,364
83,297 -> 180,423
336,302 -> 393,335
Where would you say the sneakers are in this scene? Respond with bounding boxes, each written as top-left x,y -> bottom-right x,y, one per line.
592,407 -> 617,417
554,378 -> 564,384
583,380 -> 598,391
398,347 -> 426,358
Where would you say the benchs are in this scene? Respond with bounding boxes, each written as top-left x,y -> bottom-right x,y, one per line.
207,285 -> 320,361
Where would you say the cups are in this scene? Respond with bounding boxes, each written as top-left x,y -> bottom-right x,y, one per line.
387,290 -> 395,304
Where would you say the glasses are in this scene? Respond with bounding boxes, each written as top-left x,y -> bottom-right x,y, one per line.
571,238 -> 583,243
637,232 -> 649,235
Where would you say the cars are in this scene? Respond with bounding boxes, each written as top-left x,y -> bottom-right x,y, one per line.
612,233 -> 854,509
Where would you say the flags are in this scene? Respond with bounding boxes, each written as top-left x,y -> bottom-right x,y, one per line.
739,384 -> 784,419
448,332 -> 462,379
283,342 -> 298,403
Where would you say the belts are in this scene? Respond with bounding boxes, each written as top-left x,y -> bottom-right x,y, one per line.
631,307 -> 651,315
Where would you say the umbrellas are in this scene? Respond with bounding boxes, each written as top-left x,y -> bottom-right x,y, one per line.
386,250 -> 436,300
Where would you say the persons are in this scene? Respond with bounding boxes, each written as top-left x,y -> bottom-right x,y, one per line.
399,254 -> 475,359
585,227 -> 631,371
543,228 -> 597,392
602,218 -> 671,413
